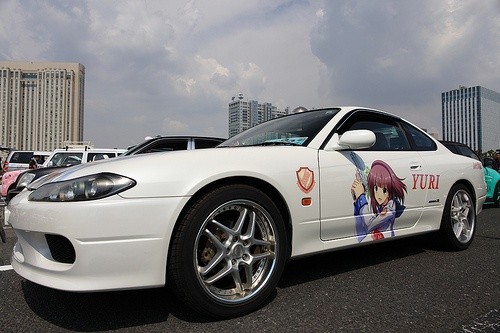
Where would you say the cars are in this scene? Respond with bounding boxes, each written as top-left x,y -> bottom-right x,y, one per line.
438,139 -> 500,205
1,107 -> 488,318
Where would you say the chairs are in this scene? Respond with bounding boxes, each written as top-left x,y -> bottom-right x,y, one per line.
363,132 -> 389,151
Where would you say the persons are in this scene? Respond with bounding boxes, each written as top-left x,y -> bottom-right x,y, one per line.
484,153 -> 500,173
29,155 -> 40,169
2,162 -> 9,175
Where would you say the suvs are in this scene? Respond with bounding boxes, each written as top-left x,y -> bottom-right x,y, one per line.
1,143 -> 130,204
6,134 -> 229,206
0,146 -> 52,173
